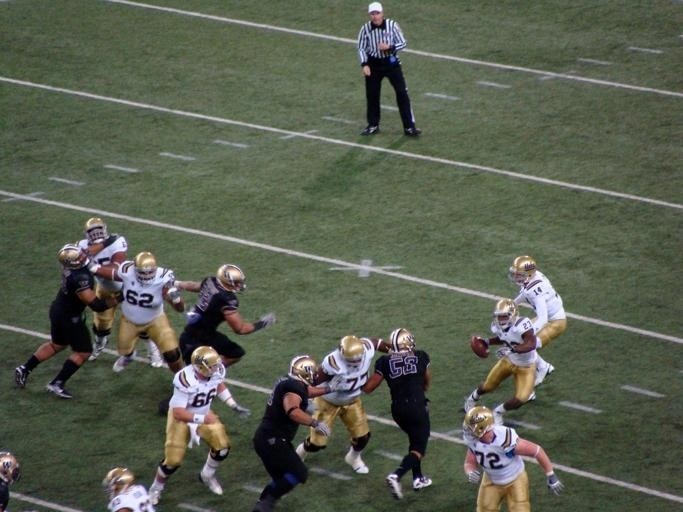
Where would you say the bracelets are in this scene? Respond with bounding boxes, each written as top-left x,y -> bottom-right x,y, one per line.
192,414 -> 205,425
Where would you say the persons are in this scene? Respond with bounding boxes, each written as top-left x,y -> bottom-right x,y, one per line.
101,466 -> 158,512
251,352 -> 348,512
354,0 -> 422,138
292,334 -> 394,475
0,449 -> 21,511
506,254 -> 570,389
464,298 -> 538,425
148,345 -> 251,506
81,249 -> 186,373
14,218 -> 162,399
358,327 -> 434,500
462,405 -> 566,511
157,263 -> 275,414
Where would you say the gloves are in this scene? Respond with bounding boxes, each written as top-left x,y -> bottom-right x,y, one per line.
547,473 -> 565,497
89,263 -> 102,277
255,313 -> 276,329
495,347 -> 510,359
313,421 -> 332,437
164,279 -> 181,305
326,375 -> 347,392
467,470 -> 480,483
225,398 -> 251,419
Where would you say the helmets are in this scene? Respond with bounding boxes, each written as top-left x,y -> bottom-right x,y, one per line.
0,452 -> 22,487
462,406 -> 493,445
508,256 -> 538,286
102,467 -> 134,503
57,216 -> 157,288
190,346 -> 226,388
288,328 -> 415,387
217,264 -> 247,294
494,298 -> 519,329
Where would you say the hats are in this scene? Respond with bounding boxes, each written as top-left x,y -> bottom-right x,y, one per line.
368,2 -> 383,13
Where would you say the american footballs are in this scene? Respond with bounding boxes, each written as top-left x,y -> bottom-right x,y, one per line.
471,335 -> 489,358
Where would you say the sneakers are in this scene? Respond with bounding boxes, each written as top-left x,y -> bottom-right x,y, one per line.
45,383 -> 72,399
464,388 -> 480,413
345,446 -> 432,500
405,127 -> 421,135
15,365 -> 29,389
199,471 -> 223,495
88,341 -> 163,372
534,363 -> 555,387
359,124 -> 379,135
296,442 -> 306,463
149,474 -> 165,505
253,499 -> 274,512
493,409 -> 504,425
